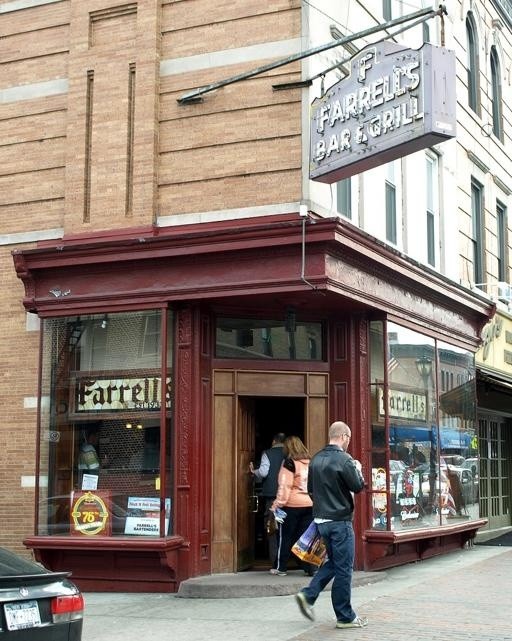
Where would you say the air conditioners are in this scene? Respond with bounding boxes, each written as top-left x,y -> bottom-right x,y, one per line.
497,281 -> 511,304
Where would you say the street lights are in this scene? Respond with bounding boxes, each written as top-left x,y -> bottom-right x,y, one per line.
415,348 -> 433,429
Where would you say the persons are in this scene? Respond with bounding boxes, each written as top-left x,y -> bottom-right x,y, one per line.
267,435 -> 316,576
294,420 -> 370,629
77,427 -> 110,471
249,431 -> 287,568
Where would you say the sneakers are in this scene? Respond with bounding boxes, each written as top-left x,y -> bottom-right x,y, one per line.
269,569 -> 288,576
305,572 -> 319,575
336,616 -> 368,629
296,591 -> 316,621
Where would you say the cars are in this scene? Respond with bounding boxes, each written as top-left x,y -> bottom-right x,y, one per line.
34,491 -> 148,541
390,455 -> 480,515
0,546 -> 84,641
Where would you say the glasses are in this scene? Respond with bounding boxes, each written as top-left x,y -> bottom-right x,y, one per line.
345,434 -> 351,440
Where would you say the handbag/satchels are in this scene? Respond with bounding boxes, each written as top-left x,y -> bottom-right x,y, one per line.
291,520 -> 327,566
